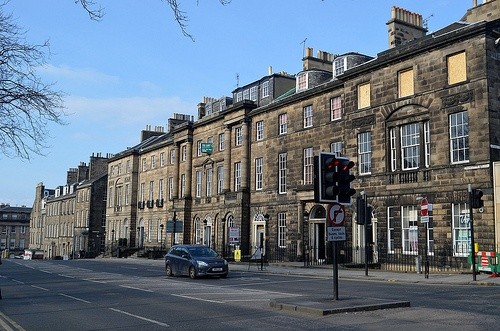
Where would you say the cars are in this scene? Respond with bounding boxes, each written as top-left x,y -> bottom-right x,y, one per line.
163,244 -> 229,279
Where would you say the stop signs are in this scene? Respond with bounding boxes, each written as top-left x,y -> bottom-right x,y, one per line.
421,198 -> 428,217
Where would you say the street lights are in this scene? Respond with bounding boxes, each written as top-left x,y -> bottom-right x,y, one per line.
160,223 -> 164,259
263,214 -> 270,266
203,218 -> 207,245
111,229 -> 115,257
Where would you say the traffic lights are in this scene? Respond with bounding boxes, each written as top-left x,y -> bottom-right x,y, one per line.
472,188 -> 484,208
335,157 -> 357,206
318,152 -> 338,203
